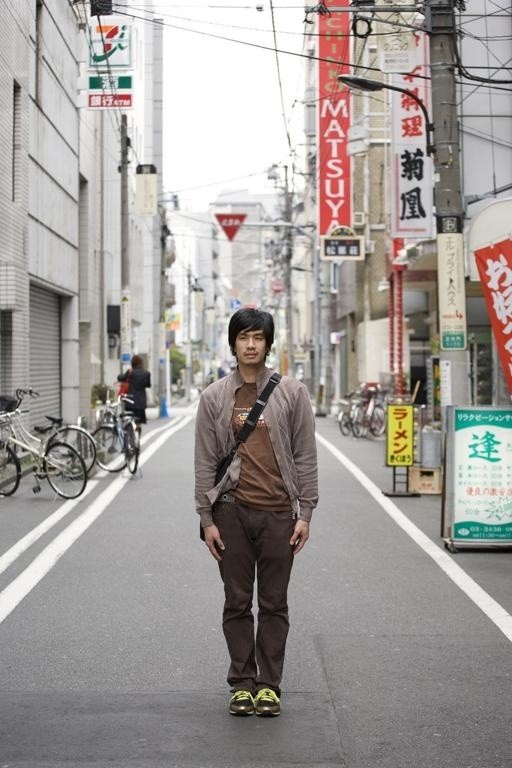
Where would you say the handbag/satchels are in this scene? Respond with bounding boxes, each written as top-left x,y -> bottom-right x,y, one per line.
199,454 -> 232,541
117,379 -> 128,395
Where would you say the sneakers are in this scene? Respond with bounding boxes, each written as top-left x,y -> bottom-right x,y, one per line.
228,687 -> 281,716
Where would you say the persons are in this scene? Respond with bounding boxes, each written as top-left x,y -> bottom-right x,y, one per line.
181,351 -> 236,396
117,355 -> 151,459
194,308 -> 319,718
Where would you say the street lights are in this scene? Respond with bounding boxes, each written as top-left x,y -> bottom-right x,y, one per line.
185,261 -> 204,401
336,74 -> 436,157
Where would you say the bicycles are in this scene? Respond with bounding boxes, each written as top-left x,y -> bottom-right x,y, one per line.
337,380 -> 387,438
0,386 -> 142,502
